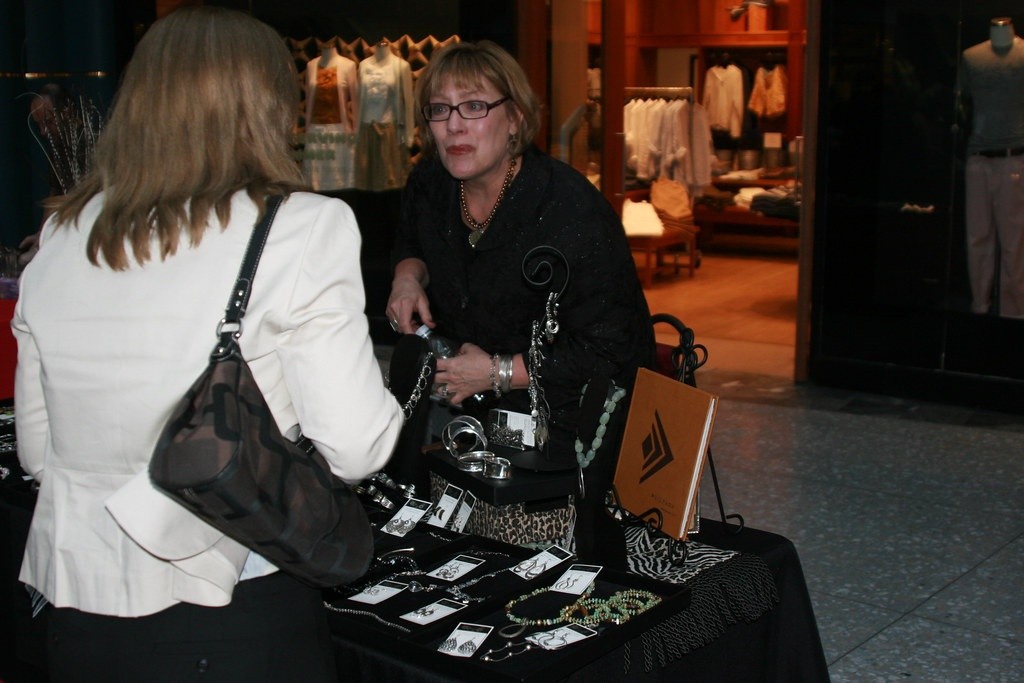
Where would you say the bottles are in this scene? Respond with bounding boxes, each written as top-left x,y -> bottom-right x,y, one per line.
415,324 -> 488,418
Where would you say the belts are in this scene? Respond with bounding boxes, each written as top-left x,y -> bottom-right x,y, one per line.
973,147 -> 1024,158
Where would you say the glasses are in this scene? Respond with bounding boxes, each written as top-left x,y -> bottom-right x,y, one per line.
420,96 -> 511,122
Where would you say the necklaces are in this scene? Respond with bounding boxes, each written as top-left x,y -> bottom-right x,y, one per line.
575,378 -> 626,468
460,159 -> 517,248
506,582 -> 593,626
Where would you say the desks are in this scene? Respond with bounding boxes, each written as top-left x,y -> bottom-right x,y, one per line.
0,404 -> 806,683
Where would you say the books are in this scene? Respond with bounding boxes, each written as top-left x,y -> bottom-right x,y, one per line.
611,367 -> 719,541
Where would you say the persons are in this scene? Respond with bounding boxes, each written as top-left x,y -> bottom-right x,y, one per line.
305,43 -> 413,191
953,18 -> 1024,320
20,78 -> 95,251
380,41 -> 658,495
9,8 -> 404,683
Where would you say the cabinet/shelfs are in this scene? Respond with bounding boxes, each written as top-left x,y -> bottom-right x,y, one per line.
695,43 -> 798,253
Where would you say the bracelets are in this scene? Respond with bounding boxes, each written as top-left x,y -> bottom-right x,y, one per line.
481,624 -> 541,661
490,353 -> 513,398
560,589 -> 662,626
441,416 -> 512,480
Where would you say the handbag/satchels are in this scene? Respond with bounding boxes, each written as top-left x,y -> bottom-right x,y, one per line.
148,185 -> 372,588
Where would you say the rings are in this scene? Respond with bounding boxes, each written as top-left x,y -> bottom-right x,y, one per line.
390,320 -> 399,331
442,385 -> 448,397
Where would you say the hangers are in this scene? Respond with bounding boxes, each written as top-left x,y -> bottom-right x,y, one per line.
624,87 -> 693,110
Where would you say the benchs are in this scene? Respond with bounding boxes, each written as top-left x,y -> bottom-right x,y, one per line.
622,222 -> 701,282
